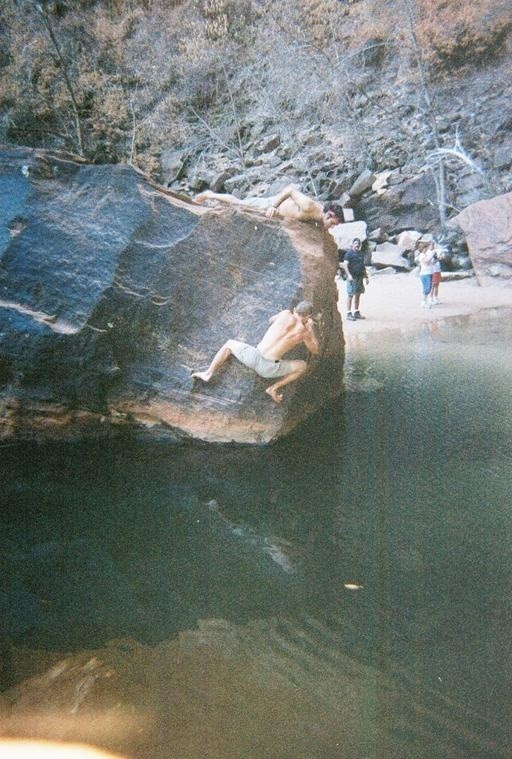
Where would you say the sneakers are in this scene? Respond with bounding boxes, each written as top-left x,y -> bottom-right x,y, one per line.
347,310 -> 366,321
423,298 -> 442,309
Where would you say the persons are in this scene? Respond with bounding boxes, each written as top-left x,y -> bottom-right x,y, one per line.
195,187 -> 344,229
418,240 -> 441,307
343,238 -> 369,320
192,301 -> 319,403
